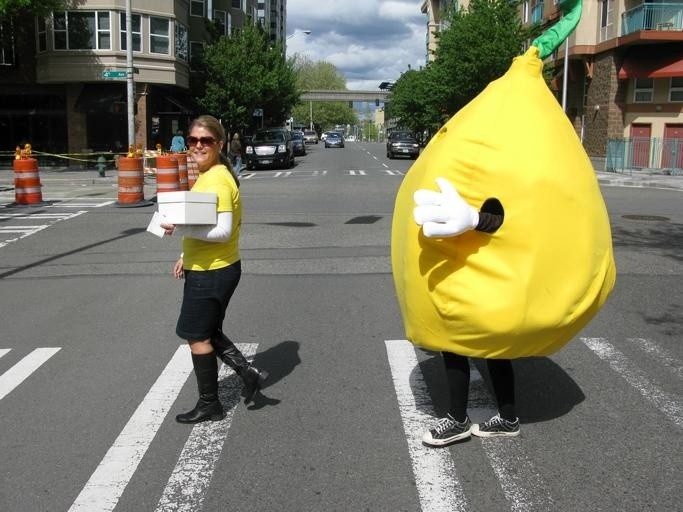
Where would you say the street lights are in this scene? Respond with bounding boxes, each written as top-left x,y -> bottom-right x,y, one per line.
284,29 -> 311,44
426,22 -> 448,30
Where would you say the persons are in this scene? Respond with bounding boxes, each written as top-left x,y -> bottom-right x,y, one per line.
166,127 -> 242,176
391,1 -> 617,447
159,115 -> 270,424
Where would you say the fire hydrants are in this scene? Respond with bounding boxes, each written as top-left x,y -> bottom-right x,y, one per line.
90,155 -> 108,179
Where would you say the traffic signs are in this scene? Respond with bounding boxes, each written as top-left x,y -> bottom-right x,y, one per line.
103,71 -> 128,78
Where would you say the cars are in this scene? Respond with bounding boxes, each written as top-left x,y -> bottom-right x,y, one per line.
320,131 -> 356,148
247,128 -> 317,172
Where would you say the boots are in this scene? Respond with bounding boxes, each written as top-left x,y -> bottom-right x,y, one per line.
211,334 -> 270,406
177,350 -> 224,423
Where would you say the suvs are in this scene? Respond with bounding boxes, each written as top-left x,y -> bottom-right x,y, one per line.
386,131 -> 421,161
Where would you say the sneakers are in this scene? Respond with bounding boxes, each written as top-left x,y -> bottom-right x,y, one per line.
472,414 -> 521,437
423,413 -> 472,445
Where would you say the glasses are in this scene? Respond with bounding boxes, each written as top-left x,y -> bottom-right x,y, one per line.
186,136 -> 218,145
